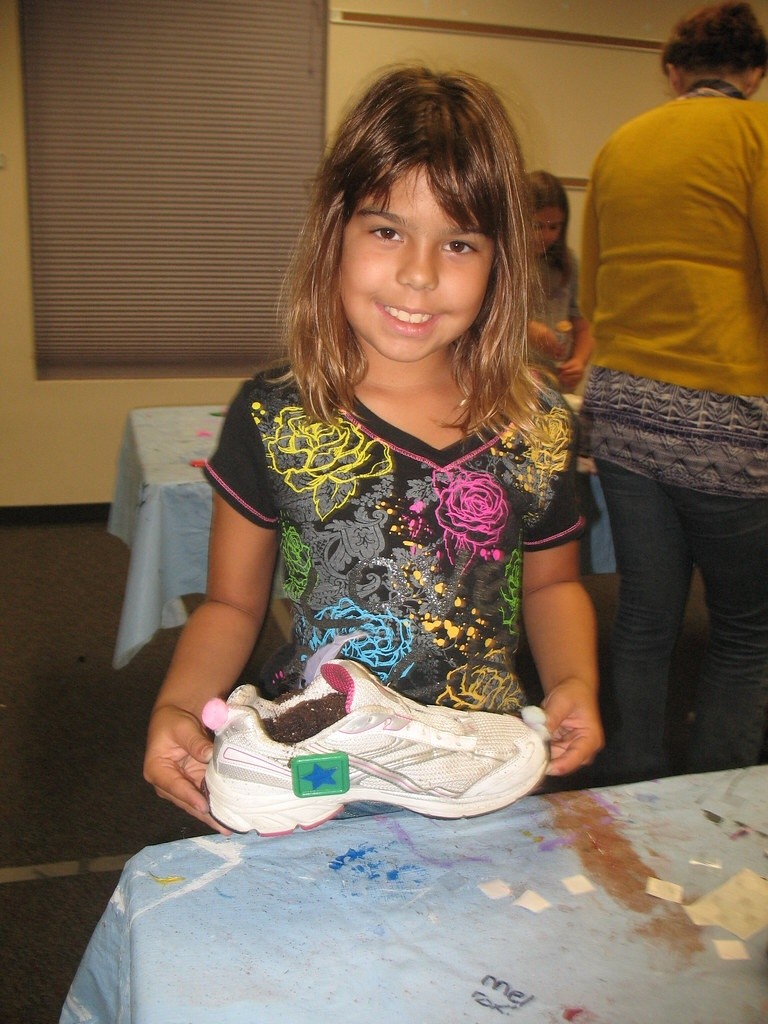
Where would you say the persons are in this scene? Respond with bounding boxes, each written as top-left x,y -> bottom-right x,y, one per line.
142,67 -> 603,839
526,170 -> 593,394
577,0 -> 768,783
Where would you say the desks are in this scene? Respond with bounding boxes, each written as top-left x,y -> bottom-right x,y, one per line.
48,752 -> 768,1022
102,397 -> 619,679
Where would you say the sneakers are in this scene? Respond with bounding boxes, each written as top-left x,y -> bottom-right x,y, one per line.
202,659 -> 548,834
